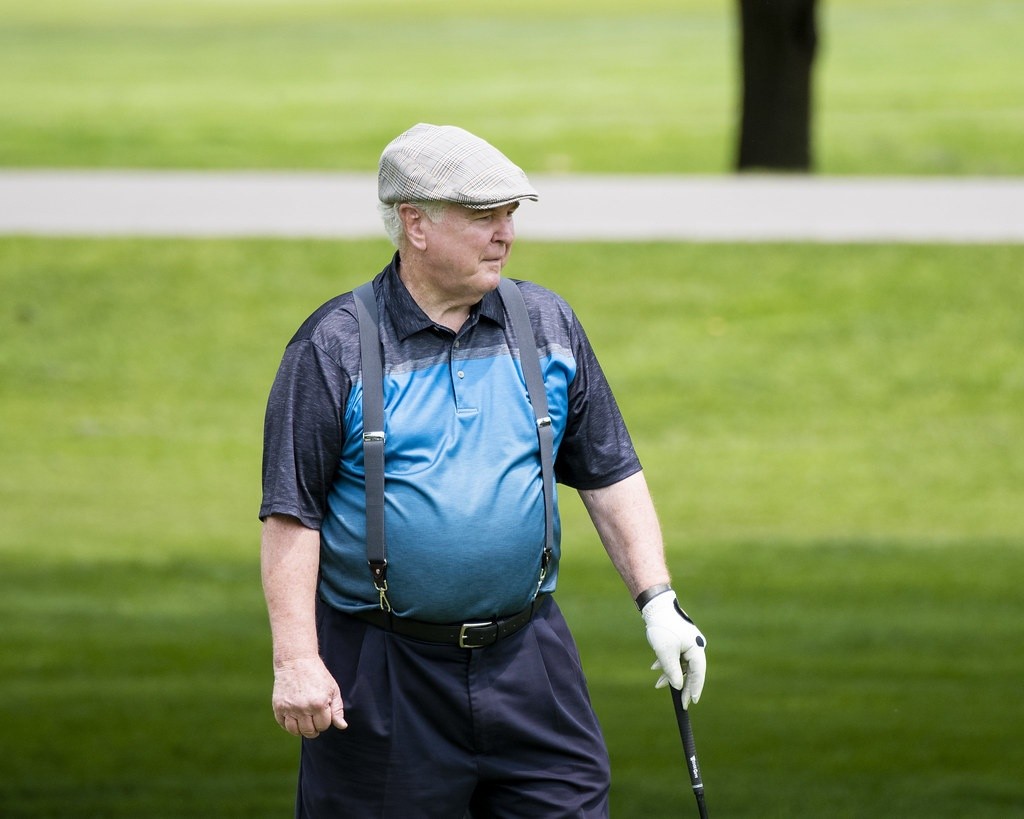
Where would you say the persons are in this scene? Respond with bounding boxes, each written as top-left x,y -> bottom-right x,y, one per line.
259,122 -> 707,818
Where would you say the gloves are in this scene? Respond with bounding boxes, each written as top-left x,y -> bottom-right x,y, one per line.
634,583 -> 706,710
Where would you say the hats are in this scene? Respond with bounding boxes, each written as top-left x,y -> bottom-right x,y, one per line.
378,123 -> 540,209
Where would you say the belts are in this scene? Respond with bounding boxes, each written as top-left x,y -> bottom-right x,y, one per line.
326,592 -> 548,649
